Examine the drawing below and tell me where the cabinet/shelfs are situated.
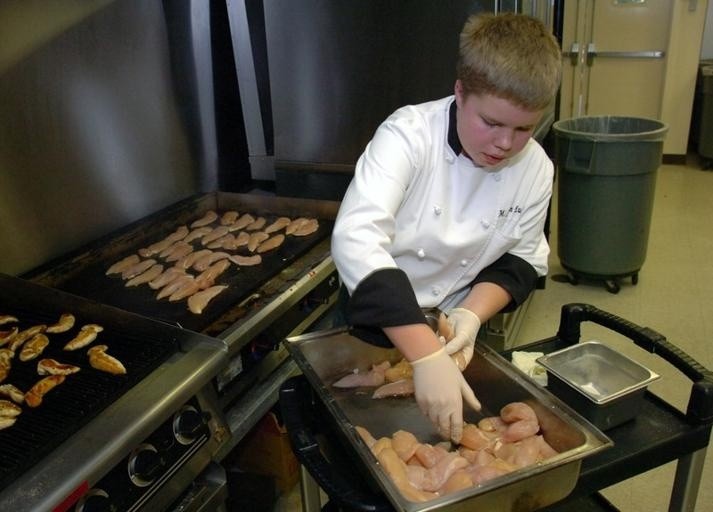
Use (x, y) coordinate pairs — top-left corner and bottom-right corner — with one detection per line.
(276, 299), (712, 510)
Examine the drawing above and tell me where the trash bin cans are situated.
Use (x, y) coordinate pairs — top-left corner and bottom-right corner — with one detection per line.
(551, 114), (668, 294)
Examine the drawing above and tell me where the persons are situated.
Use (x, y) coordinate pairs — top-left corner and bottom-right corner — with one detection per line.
(329, 12), (562, 443)
